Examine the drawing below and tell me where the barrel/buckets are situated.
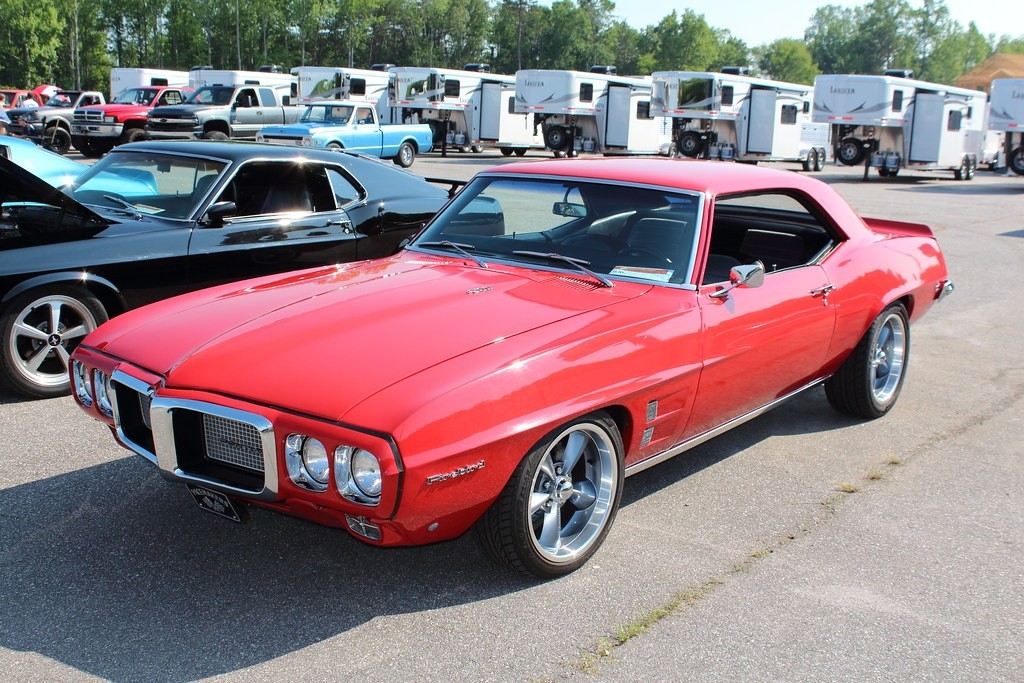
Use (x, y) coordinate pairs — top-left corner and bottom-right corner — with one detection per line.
(445, 132), (465, 145)
(574, 137), (593, 152)
(710, 145), (734, 159)
(871, 151), (898, 169)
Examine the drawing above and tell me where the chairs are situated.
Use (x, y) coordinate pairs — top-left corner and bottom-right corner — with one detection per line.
(734, 229), (804, 274)
(190, 173), (236, 204)
(626, 217), (688, 265)
(259, 179), (313, 213)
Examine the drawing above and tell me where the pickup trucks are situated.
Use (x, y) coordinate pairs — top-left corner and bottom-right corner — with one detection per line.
(256, 101), (432, 168)
(144, 84), (332, 141)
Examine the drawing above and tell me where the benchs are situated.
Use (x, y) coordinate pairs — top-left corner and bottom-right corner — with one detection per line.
(586, 211), (828, 272)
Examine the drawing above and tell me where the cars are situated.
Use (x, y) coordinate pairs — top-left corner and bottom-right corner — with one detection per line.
(1, 135), (159, 197)
(0, 140), (504, 399)
(69, 156), (955, 579)
(0, 90), (70, 111)
(70, 86), (201, 158)
(3, 91), (107, 153)
